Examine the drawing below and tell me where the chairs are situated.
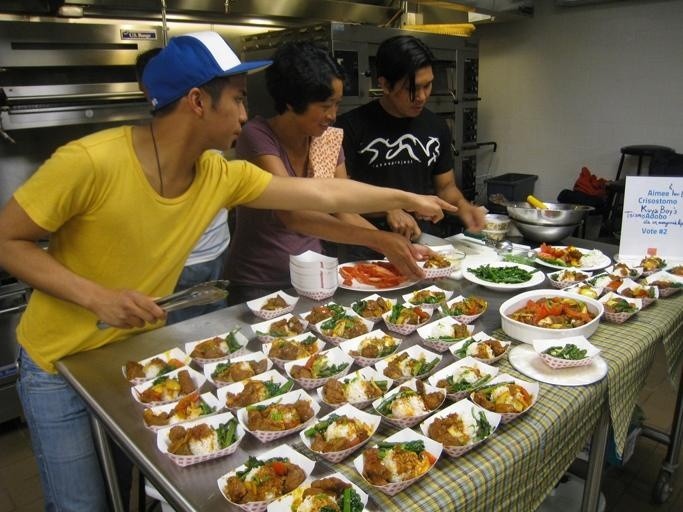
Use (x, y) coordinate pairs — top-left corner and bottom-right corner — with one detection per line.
(597, 144), (676, 245)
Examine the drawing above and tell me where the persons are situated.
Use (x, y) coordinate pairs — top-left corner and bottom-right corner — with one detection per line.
(0, 32), (458, 512)
(335, 35), (485, 242)
(148, 120), (163, 197)
(135, 48), (231, 326)
(224, 41), (442, 306)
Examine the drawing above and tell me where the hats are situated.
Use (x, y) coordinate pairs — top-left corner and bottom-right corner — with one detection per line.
(141, 31), (274, 110)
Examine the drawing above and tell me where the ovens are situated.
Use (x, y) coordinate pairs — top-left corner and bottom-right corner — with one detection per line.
(240, 22), (479, 236)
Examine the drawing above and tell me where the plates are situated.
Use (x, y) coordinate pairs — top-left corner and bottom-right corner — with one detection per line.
(463, 262), (546, 292)
(338, 260), (424, 292)
(528, 246), (611, 271)
(508, 343), (608, 386)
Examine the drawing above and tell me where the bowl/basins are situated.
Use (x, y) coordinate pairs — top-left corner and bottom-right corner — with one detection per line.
(501, 201), (595, 225)
(481, 230), (511, 242)
(508, 217), (584, 244)
(485, 214), (511, 230)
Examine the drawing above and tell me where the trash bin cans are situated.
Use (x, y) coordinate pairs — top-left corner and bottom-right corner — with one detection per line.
(484, 173), (538, 213)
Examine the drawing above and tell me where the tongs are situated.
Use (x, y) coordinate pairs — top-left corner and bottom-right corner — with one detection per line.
(97, 280), (230, 331)
